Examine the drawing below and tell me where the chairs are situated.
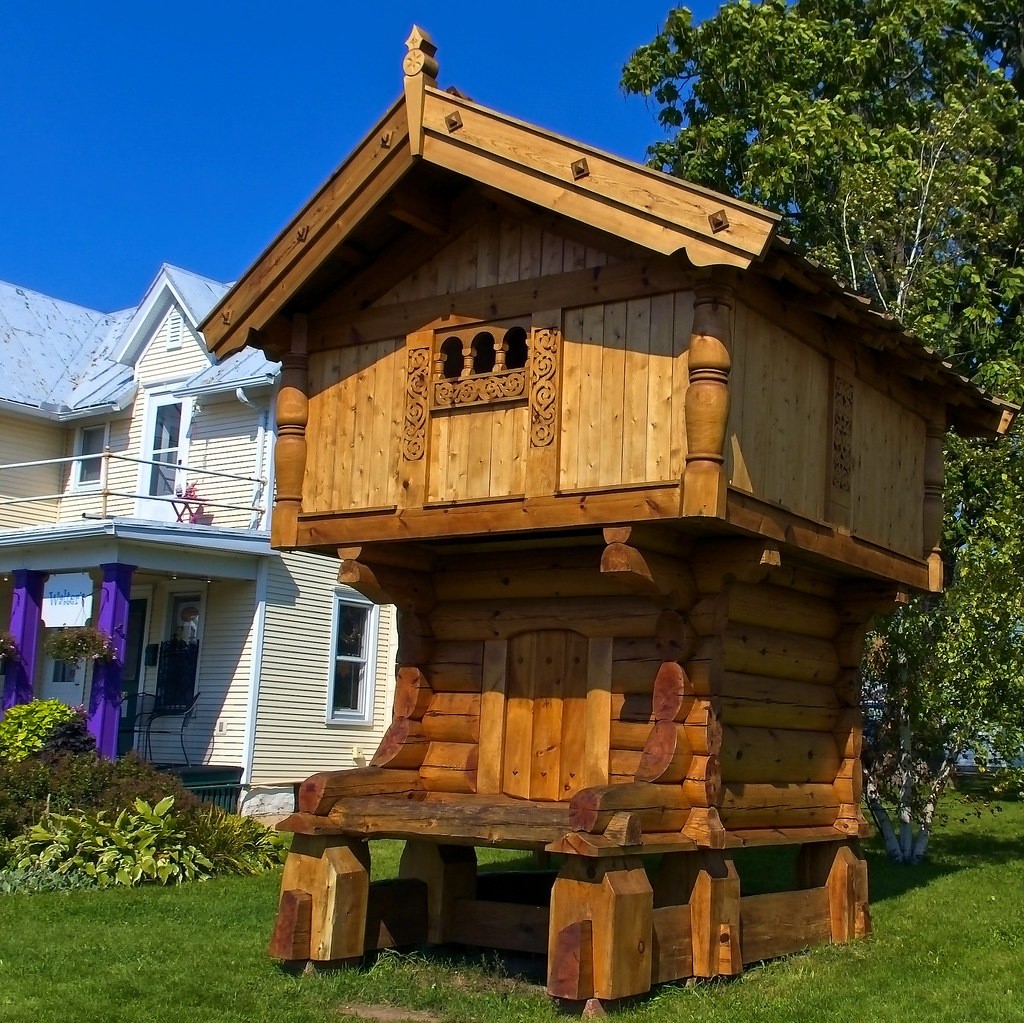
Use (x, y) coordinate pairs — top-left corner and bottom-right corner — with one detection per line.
(143, 691), (203, 767)
(117, 691), (157, 765)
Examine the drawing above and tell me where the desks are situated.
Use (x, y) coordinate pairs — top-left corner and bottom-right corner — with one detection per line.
(165, 498), (208, 528)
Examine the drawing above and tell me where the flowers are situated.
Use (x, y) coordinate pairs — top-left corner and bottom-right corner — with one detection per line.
(43, 627), (118, 663)
(0, 631), (18, 660)
